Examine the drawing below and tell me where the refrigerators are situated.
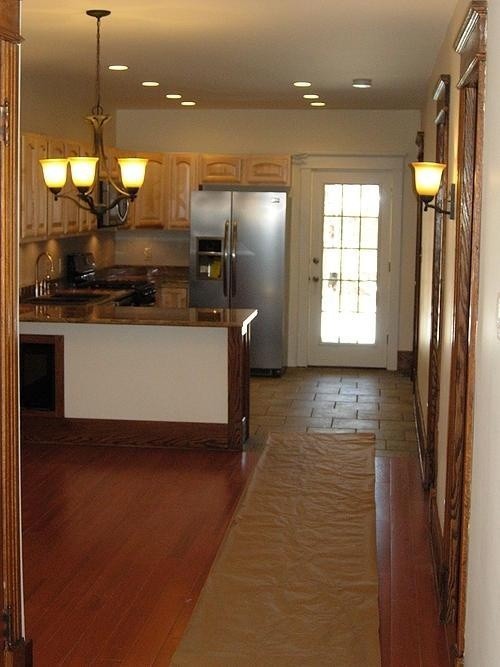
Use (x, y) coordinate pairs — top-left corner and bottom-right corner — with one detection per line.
(188, 189), (289, 378)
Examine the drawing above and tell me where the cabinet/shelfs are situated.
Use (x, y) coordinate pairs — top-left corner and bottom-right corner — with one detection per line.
(118, 151), (167, 230)
(65, 139), (120, 236)
(20, 135), (63, 244)
(167, 153), (200, 232)
(201, 153), (291, 185)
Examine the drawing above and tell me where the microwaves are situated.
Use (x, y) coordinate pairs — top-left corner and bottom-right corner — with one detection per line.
(100, 181), (129, 227)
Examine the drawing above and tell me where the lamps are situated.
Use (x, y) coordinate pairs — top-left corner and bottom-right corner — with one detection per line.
(38, 10), (148, 228)
(409, 160), (455, 219)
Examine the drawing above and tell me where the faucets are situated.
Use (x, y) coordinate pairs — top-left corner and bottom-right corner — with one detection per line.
(34, 252), (55, 296)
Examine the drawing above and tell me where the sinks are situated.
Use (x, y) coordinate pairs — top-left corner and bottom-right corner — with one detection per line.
(22, 293), (111, 305)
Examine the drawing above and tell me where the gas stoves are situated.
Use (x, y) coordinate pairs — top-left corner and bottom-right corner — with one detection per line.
(78, 279), (157, 304)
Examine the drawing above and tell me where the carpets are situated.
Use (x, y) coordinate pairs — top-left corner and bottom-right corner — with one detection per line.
(165, 432), (383, 665)
